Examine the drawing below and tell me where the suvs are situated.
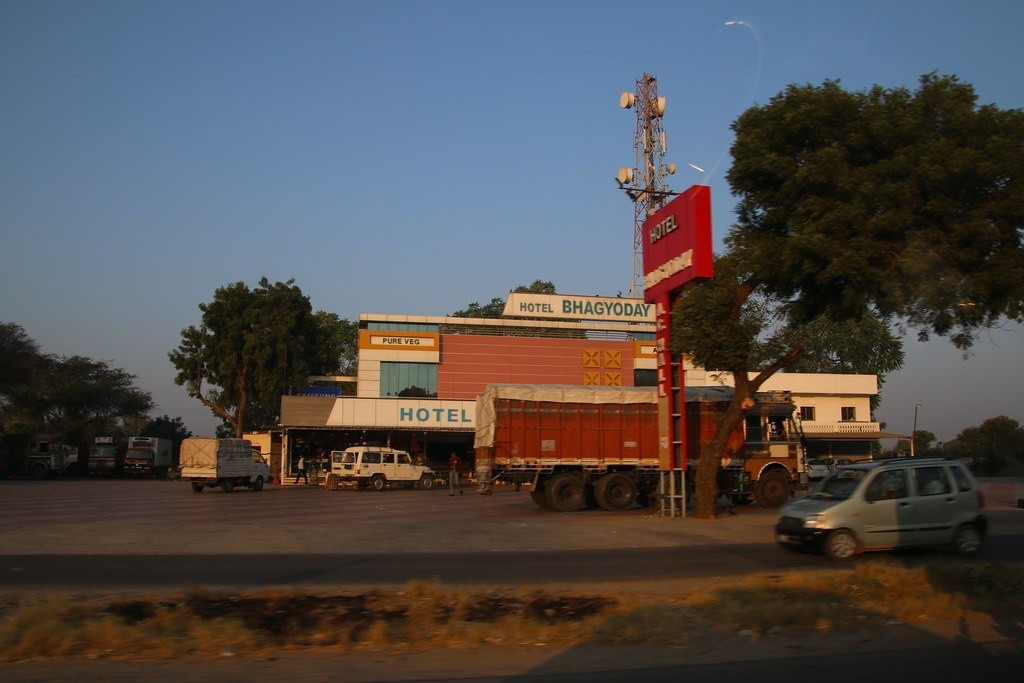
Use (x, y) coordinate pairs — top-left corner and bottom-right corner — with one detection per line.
(330, 446), (434, 492)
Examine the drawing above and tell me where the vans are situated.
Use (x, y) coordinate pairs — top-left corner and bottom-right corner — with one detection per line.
(775, 455), (990, 561)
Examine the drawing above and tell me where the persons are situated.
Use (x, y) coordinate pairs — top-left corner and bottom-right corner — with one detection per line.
(448, 452), (464, 497)
(295, 454), (309, 484)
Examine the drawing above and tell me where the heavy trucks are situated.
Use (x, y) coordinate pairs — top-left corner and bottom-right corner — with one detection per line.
(473, 380), (808, 514)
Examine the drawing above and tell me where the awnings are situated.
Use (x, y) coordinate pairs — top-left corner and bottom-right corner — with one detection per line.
(802, 432), (916, 459)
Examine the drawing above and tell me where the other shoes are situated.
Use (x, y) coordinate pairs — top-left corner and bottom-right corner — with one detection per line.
(460, 489), (463, 494)
(450, 494), (454, 496)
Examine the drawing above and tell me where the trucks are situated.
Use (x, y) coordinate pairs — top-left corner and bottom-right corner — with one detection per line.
(179, 437), (271, 493)
(88, 437), (121, 478)
(124, 436), (173, 479)
(21, 441), (78, 475)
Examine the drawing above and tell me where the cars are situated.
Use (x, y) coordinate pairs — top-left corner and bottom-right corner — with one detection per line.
(807, 457), (859, 480)
